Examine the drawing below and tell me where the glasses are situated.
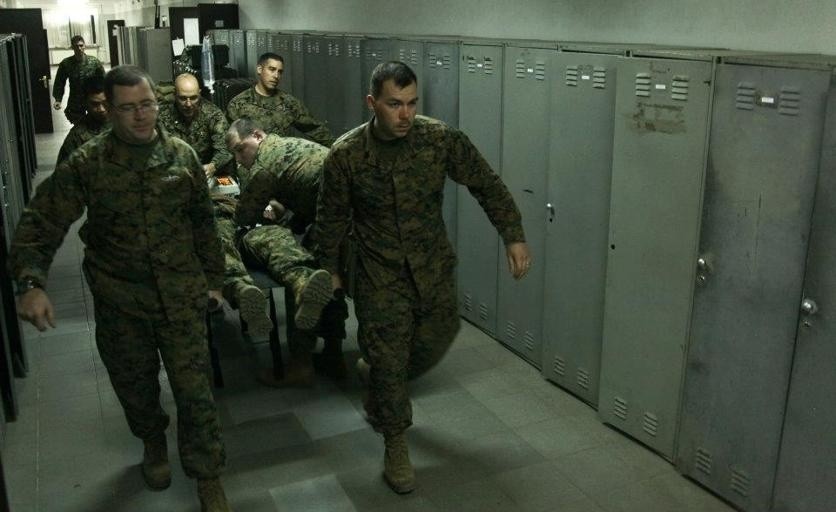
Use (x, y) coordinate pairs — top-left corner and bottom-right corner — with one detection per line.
(110, 101), (160, 114)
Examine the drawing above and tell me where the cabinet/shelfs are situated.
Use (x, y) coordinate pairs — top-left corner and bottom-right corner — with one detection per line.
(0, 5), (60, 504)
(205, 26), (836, 509)
(115, 26), (172, 85)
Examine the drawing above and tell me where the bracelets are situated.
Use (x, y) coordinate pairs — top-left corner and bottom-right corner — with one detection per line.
(265, 204), (275, 211)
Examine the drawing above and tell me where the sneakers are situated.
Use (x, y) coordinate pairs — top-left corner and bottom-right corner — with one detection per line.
(292, 269), (335, 331)
(233, 281), (273, 337)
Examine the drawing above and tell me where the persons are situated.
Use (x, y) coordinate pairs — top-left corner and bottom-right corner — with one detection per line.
(225, 52), (337, 148)
(54, 82), (110, 165)
(6, 66), (228, 511)
(51, 33), (104, 124)
(157, 70), (232, 177)
(225, 115), (375, 384)
(210, 192), (336, 348)
(310, 60), (532, 495)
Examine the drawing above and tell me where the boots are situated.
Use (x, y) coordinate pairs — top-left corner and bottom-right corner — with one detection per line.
(141, 432), (172, 491)
(196, 476), (229, 511)
(384, 432), (417, 495)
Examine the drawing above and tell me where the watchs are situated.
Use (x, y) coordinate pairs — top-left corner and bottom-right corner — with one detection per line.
(17, 280), (45, 293)
